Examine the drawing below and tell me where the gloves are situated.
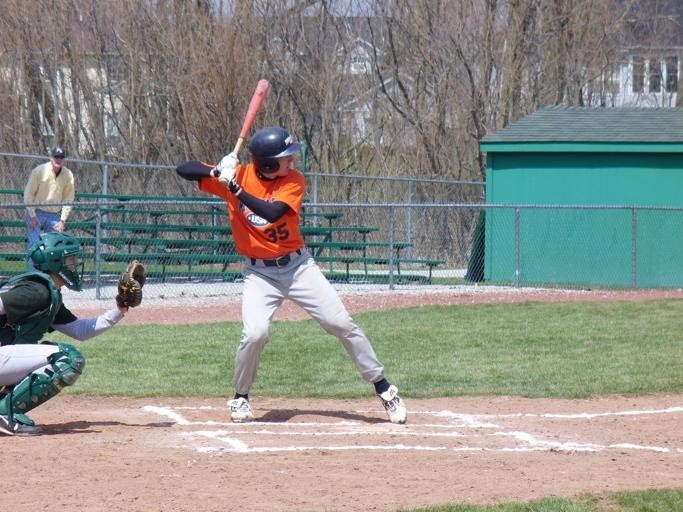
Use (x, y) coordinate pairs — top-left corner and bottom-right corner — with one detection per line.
(210, 152), (240, 192)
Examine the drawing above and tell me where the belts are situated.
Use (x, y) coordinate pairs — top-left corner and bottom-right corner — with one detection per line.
(251, 249), (303, 267)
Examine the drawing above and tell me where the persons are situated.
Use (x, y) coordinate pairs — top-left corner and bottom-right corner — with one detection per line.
(174, 124), (407, 424)
(23, 146), (76, 272)
(0, 231), (146, 436)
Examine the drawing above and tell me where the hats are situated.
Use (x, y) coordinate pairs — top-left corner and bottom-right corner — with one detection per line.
(53, 148), (65, 157)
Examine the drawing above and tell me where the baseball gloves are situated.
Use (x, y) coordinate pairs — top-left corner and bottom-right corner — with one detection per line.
(116, 261), (145, 308)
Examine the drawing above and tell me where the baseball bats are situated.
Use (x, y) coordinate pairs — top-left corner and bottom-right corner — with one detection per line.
(219, 80), (272, 186)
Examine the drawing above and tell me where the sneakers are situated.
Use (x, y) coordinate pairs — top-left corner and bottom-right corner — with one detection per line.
(0, 414), (43, 436)
(228, 397), (254, 424)
(375, 383), (407, 423)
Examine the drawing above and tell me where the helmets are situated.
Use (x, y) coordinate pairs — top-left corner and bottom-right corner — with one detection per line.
(25, 232), (84, 292)
(248, 128), (302, 173)
